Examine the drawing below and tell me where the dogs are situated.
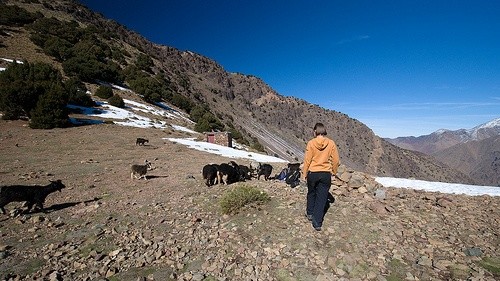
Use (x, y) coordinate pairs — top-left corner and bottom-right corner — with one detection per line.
(136, 138), (149, 146)
(202, 160), (301, 187)
(0, 180), (67, 216)
(131, 159), (153, 181)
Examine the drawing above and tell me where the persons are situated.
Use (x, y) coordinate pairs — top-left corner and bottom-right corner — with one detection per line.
(303, 123), (339, 230)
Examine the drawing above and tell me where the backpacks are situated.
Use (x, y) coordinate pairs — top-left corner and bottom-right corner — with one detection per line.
(286, 170), (301, 188)
(278, 168), (290, 180)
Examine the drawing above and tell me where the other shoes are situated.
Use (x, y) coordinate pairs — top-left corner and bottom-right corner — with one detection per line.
(312, 221), (321, 230)
(305, 210), (313, 221)
